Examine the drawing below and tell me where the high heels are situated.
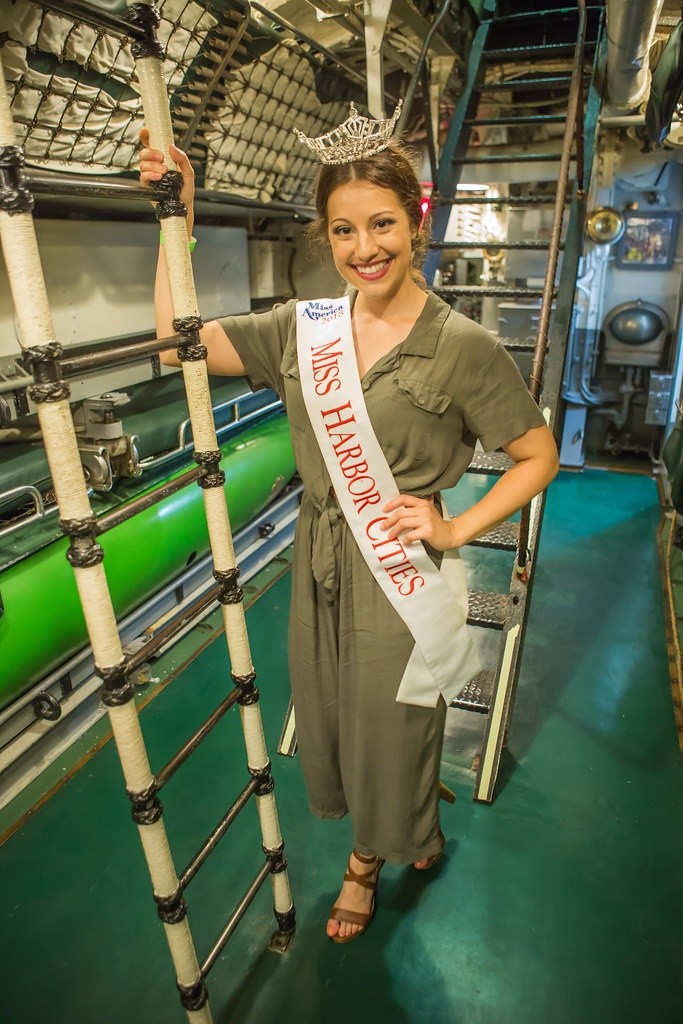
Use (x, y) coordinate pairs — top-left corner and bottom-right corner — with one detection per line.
(415, 782), (456, 868)
(330, 848), (385, 944)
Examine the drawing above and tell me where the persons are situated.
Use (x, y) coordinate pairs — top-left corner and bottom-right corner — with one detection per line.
(136, 99), (560, 943)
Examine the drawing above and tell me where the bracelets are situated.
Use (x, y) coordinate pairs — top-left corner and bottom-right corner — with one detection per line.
(159, 227), (197, 254)
